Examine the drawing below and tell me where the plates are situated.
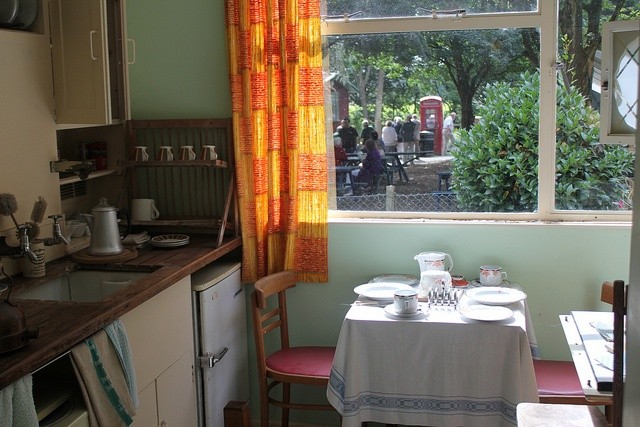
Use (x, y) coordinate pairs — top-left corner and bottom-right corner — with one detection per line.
(354, 282), (414, 301)
(373, 274), (419, 285)
(466, 286), (528, 305)
(458, 305), (512, 321)
(151, 235), (190, 248)
(470, 277), (511, 287)
(385, 305), (422, 317)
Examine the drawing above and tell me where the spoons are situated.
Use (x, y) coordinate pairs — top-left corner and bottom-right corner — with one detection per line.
(350, 302), (386, 309)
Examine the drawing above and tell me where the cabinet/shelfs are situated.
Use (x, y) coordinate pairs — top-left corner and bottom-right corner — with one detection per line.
(31, 0), (131, 123)
(55, 129), (125, 222)
(129, 348), (199, 427)
(115, 118), (239, 248)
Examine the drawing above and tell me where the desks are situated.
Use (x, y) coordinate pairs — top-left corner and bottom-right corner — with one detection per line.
(336, 151), (434, 182)
(336, 165), (361, 195)
(436, 172), (451, 209)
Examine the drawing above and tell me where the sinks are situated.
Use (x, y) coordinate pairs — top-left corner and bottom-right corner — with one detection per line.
(9, 261), (165, 302)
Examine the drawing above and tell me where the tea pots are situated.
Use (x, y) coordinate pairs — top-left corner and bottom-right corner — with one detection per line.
(414, 251), (453, 294)
(77, 197), (125, 255)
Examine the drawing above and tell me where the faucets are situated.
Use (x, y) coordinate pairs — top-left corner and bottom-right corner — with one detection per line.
(0, 223), (39, 263)
(36, 214), (70, 247)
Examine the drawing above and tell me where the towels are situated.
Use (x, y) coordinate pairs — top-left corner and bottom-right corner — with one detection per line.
(69, 329), (136, 427)
(102, 318), (139, 408)
(0, 373), (39, 427)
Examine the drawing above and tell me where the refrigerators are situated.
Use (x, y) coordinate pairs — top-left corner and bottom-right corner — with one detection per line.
(192, 264), (249, 426)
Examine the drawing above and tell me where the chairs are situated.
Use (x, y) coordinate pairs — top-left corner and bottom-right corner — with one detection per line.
(532, 281), (626, 424)
(250, 270), (342, 427)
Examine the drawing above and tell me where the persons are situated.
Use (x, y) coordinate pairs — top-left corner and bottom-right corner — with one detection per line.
(443, 112), (458, 153)
(353, 139), (383, 195)
(398, 115), (416, 164)
(333, 136), (348, 196)
(381, 121), (398, 155)
(412, 114), (424, 159)
(392, 117), (398, 125)
(336, 117), (358, 152)
(363, 131), (384, 152)
(360, 119), (377, 146)
(394, 119), (404, 150)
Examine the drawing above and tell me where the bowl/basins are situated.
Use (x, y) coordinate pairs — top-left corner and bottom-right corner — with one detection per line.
(65, 219), (87, 238)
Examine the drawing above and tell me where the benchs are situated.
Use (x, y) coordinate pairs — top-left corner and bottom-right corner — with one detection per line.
(386, 157), (410, 182)
(337, 157), (394, 184)
(336, 174), (383, 196)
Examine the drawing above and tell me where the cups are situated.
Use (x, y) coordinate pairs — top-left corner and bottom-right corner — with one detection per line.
(21, 241), (46, 278)
(421, 272), (451, 296)
(134, 146), (150, 161)
(480, 265), (507, 286)
(394, 289), (418, 314)
(180, 146), (196, 161)
(131, 198), (159, 222)
(202, 145), (217, 160)
(159, 146), (174, 161)
